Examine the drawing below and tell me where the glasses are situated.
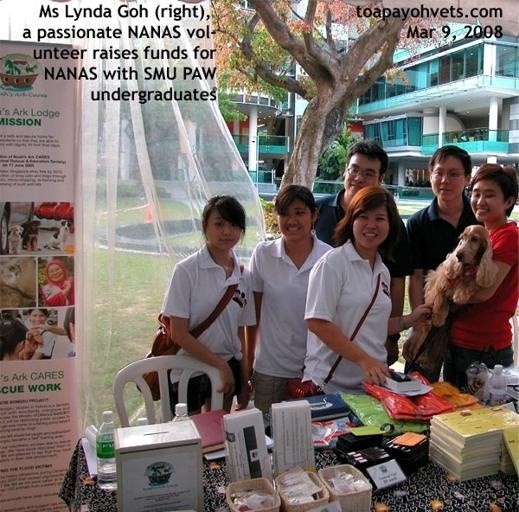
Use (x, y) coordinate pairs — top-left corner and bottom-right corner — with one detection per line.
(346, 166), (381, 179)
(431, 170), (466, 178)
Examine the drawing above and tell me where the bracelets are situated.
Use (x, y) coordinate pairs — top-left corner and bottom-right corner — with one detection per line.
(398, 317), (404, 330)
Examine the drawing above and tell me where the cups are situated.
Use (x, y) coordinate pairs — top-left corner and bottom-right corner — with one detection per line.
(465, 358), (490, 403)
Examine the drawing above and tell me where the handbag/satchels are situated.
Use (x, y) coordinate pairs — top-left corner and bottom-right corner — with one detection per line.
(286, 377), (319, 401)
(135, 312), (182, 402)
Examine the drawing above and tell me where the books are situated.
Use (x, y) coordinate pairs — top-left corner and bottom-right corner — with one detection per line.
(429, 399), (517, 488)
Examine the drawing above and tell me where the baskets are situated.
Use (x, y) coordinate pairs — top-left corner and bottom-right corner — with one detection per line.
(272, 469), (330, 511)
(224, 477), (281, 511)
(317, 463), (373, 511)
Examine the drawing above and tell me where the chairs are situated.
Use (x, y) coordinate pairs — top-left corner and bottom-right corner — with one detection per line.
(109, 355), (223, 426)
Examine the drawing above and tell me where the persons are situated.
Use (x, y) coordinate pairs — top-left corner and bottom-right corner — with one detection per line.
(436, 161), (518, 389)
(246, 182), (337, 411)
(398, 143), (489, 361)
(40, 260), (74, 306)
(299, 186), (429, 400)
(158, 195), (258, 415)
(0, 307), (76, 362)
(310, 138), (408, 368)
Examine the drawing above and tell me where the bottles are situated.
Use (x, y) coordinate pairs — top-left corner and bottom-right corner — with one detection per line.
(171, 402), (191, 423)
(488, 364), (508, 407)
(96, 410), (119, 491)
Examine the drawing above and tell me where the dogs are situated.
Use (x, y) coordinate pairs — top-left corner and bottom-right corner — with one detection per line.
(3, 329), (43, 360)
(21, 220), (42, 251)
(8, 225), (25, 255)
(43, 220), (72, 251)
(409, 224), (496, 356)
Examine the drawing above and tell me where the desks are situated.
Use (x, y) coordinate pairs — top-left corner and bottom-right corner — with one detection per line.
(77, 385), (519, 512)
